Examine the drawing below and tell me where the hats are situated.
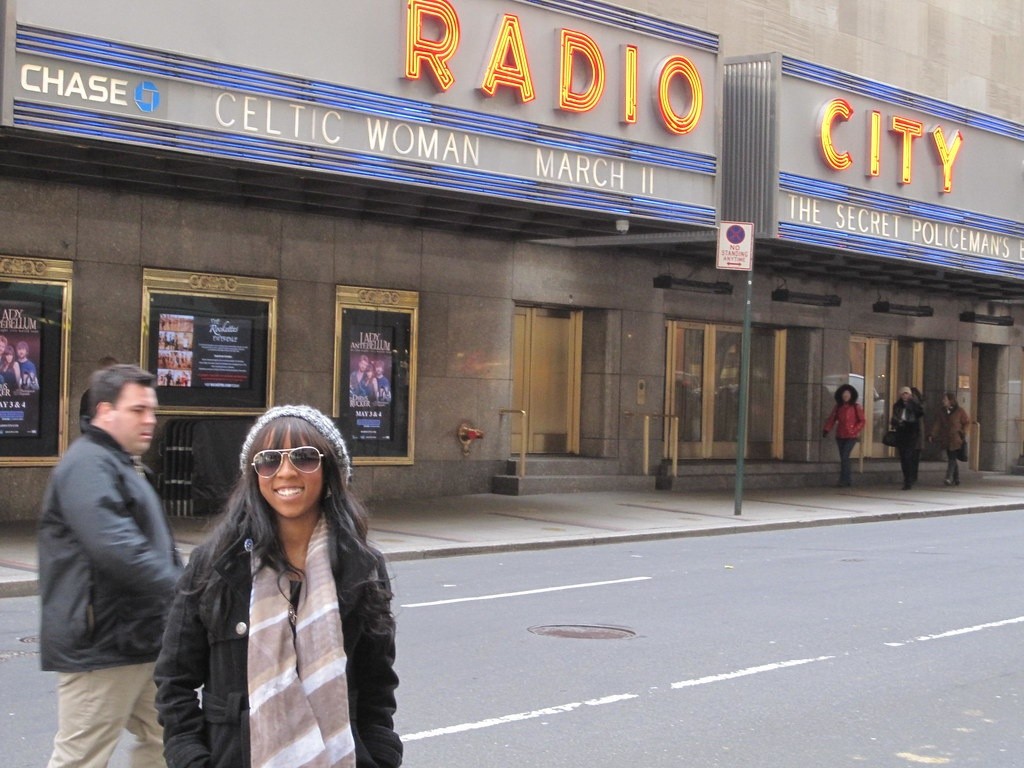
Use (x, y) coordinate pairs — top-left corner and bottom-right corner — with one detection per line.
(899, 384), (911, 396)
(240, 404), (351, 487)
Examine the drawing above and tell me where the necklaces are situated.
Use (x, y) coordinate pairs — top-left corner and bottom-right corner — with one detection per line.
(286, 602), (298, 626)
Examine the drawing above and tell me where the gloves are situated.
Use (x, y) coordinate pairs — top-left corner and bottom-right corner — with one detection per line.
(823, 429), (828, 439)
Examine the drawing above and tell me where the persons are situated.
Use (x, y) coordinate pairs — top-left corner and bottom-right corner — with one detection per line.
(823, 384), (865, 486)
(158, 319), (188, 386)
(928, 393), (970, 486)
(890, 386), (925, 490)
(154, 404), (405, 768)
(349, 355), (391, 407)
(39, 364), (185, 768)
(80, 356), (119, 433)
(0, 335), (37, 390)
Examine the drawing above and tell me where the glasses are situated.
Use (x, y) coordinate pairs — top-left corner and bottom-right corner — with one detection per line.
(251, 446), (324, 480)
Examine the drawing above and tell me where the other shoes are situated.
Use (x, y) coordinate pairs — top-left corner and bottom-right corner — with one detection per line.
(945, 478), (960, 486)
(902, 481), (912, 491)
(836, 480), (852, 487)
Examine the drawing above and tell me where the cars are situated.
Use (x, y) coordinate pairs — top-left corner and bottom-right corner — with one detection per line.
(677, 371), (769, 400)
(822, 375), (886, 419)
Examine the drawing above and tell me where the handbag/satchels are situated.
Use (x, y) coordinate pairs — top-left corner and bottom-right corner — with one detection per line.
(956, 440), (968, 462)
(883, 422), (900, 448)
